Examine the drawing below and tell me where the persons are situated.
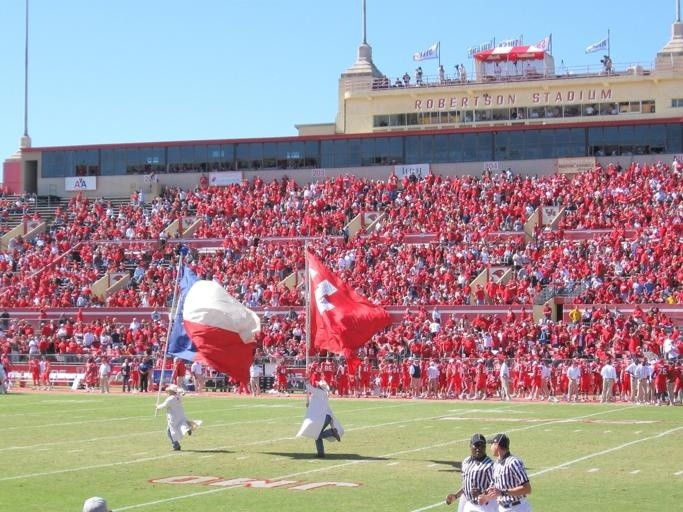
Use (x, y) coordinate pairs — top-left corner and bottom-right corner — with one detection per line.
(494, 64), (502, 80)
(600, 55), (613, 75)
(446, 434), (496, 512)
(373, 68), (423, 86)
(83, 496), (109, 510)
(296, 372), (343, 459)
(438, 64), (467, 84)
(488, 434), (532, 512)
(463, 102), (619, 121)
(155, 384), (200, 452)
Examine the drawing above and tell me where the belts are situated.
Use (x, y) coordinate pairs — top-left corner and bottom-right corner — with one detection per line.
(471, 500), (488, 506)
(503, 501), (521, 508)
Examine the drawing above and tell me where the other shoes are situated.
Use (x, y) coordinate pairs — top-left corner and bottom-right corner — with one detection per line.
(332, 392), (682, 408)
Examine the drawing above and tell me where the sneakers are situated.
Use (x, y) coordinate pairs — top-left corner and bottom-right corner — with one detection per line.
(315, 454), (325, 458)
(333, 428), (340, 442)
(33, 387), (150, 393)
(195, 389), (307, 397)
(187, 430), (191, 435)
(170, 447), (181, 451)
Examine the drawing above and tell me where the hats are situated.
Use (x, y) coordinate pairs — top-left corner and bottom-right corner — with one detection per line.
(489, 434), (510, 447)
(310, 304), (683, 370)
(470, 434), (486, 445)
(165, 384), (178, 392)
(315, 380), (329, 390)
(82, 496), (113, 512)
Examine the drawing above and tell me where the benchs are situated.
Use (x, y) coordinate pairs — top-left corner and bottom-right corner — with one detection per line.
(0, 191), (195, 307)
(4, 368), (87, 393)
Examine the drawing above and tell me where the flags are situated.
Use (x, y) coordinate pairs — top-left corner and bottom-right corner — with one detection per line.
(586, 36), (609, 54)
(413, 43), (438, 61)
(467, 40), (494, 57)
(499, 38), (520, 47)
(536, 36), (549, 50)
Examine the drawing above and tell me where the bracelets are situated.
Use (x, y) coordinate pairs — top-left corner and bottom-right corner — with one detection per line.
(455, 494), (458, 500)
(501, 489), (508, 497)
